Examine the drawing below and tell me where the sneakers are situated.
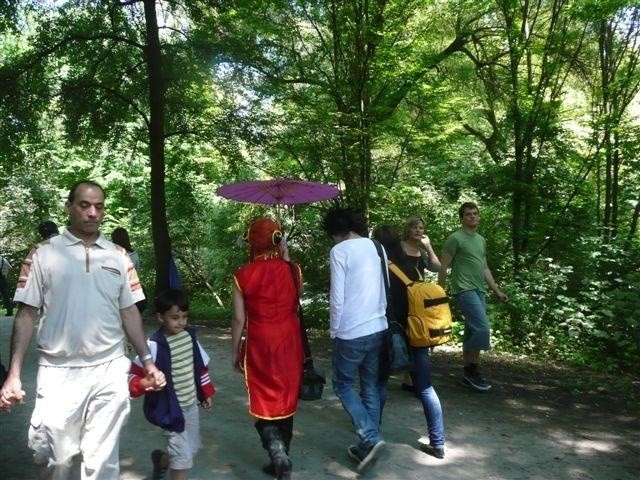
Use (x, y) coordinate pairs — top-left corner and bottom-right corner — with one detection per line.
(355, 439), (387, 476)
(401, 381), (416, 392)
(152, 447), (170, 480)
(347, 443), (361, 462)
(460, 361), (493, 391)
(420, 443), (446, 458)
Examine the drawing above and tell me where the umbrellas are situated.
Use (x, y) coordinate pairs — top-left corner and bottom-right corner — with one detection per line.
(216, 179), (339, 230)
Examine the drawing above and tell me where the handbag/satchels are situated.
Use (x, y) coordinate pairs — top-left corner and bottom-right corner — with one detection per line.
(385, 317), (414, 371)
(297, 361), (326, 401)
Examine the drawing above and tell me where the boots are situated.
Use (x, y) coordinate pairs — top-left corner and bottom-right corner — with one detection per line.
(253, 416), (293, 480)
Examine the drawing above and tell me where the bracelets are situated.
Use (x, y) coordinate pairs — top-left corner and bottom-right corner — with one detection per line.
(139, 354), (153, 363)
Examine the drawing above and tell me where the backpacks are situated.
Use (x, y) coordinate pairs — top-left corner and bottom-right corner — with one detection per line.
(388, 258), (454, 347)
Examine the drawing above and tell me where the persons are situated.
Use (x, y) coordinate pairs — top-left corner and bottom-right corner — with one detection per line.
(323, 207), (390, 474)
(38, 221), (60, 241)
(112, 226), (139, 274)
(128, 288), (216, 479)
(0, 179), (162, 479)
(373, 226), (445, 457)
(0, 251), (14, 316)
(399, 217), (441, 393)
(439, 202), (507, 391)
(230, 219), (304, 479)
(0, 360), (25, 415)
